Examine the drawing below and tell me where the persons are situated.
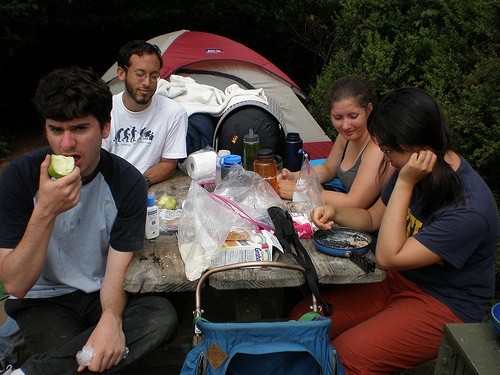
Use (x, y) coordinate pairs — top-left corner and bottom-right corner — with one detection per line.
(277, 76), (397, 210)
(100, 40), (188, 190)
(287, 88), (500, 375)
(0, 66), (180, 375)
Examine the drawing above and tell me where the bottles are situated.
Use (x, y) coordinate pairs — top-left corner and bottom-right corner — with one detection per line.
(253, 149), (278, 194)
(215, 150), (230, 186)
(144, 196), (159, 239)
(219, 155), (243, 182)
(283, 133), (303, 172)
(244, 129), (260, 171)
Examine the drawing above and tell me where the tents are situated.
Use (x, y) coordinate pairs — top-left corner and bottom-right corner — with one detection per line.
(101, 29), (334, 161)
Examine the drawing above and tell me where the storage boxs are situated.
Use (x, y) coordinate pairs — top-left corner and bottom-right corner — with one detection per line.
(209, 229), (281, 269)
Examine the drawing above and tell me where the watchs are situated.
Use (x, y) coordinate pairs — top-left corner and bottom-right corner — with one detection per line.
(143, 175), (150, 189)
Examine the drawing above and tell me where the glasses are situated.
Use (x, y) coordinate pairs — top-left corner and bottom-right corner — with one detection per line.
(379, 141), (404, 155)
(124, 64), (162, 83)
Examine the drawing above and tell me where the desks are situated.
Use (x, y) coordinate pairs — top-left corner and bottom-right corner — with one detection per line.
(122, 167), (387, 324)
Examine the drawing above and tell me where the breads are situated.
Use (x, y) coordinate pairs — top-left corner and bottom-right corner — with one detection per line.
(225, 231), (247, 241)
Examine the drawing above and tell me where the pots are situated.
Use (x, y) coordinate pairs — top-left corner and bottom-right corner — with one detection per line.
(312, 227), (376, 274)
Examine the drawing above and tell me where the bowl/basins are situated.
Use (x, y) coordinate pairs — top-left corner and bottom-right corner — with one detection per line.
(490, 302), (500, 337)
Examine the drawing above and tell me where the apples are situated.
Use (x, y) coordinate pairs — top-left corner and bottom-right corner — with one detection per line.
(156, 195), (176, 210)
(48, 154), (75, 179)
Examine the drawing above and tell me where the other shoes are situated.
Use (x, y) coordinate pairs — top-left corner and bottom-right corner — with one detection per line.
(0, 330), (25, 370)
